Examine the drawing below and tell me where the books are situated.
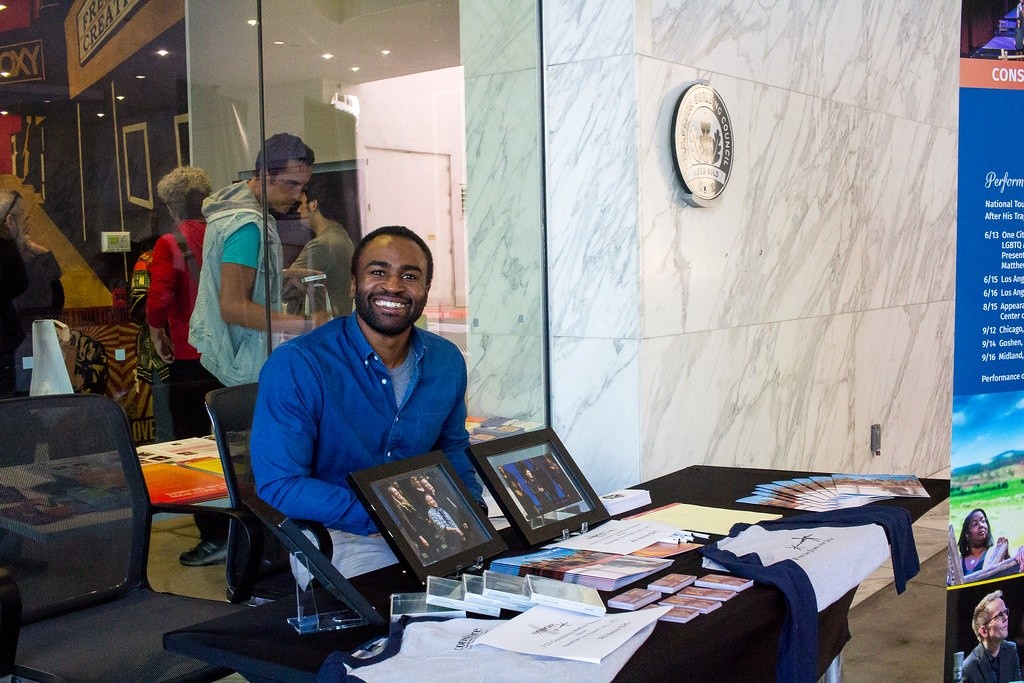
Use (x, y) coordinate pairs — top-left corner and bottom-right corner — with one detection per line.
(465, 415), (545, 445)
(0, 429), (251, 539)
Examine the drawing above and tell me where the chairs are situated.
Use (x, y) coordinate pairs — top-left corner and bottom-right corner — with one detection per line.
(0, 393), (258, 683)
(204, 382), (336, 605)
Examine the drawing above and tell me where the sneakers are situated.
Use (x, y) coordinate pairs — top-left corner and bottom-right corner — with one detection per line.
(178, 539), (230, 566)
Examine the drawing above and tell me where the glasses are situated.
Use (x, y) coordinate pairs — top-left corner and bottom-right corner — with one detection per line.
(983, 608), (1010, 626)
(967, 518), (988, 529)
(2, 189), (24, 222)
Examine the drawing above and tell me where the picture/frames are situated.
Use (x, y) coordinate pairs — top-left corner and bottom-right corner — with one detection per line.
(344, 449), (510, 587)
(464, 426), (612, 548)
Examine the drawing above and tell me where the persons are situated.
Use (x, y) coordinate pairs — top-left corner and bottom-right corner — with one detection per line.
(947, 509), (1020, 587)
(128, 132), (356, 565)
(0, 192), (65, 683)
(388, 474), (479, 561)
(496, 456), (581, 518)
(247, 226), (487, 592)
(962, 589), (1021, 683)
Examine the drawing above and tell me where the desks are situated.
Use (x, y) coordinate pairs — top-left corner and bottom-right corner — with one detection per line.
(161, 465), (950, 683)
